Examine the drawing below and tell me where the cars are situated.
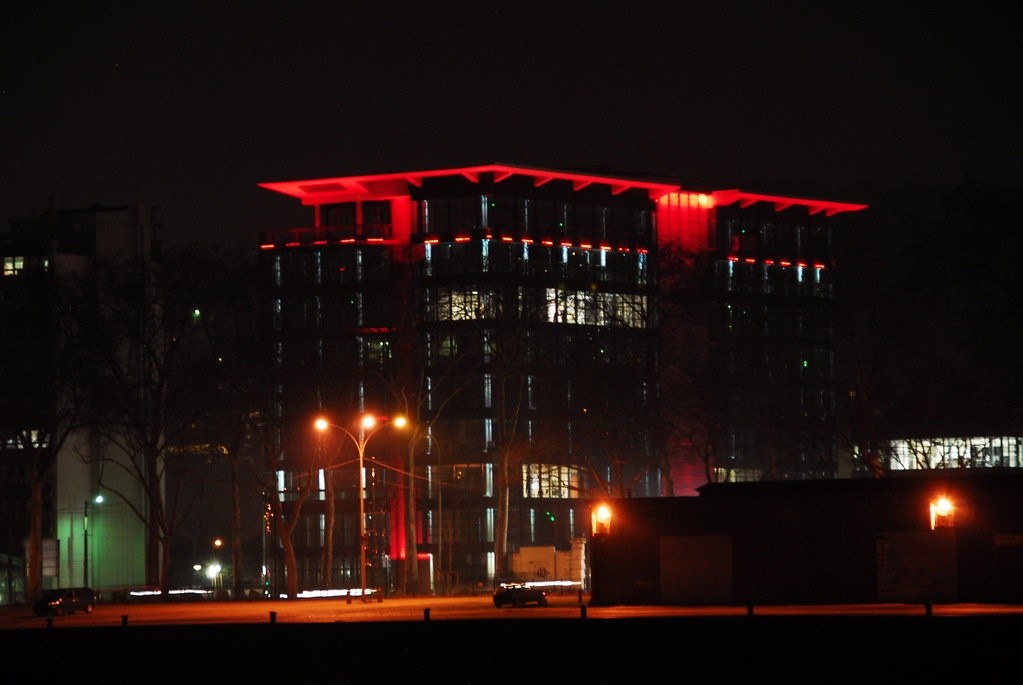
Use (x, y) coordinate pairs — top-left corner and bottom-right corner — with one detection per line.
(492, 585), (546, 609)
(33, 587), (98, 618)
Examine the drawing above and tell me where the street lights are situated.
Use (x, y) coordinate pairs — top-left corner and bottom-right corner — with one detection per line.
(591, 504), (611, 534)
(84, 495), (102, 592)
(315, 412), (410, 598)
(927, 499), (954, 531)
